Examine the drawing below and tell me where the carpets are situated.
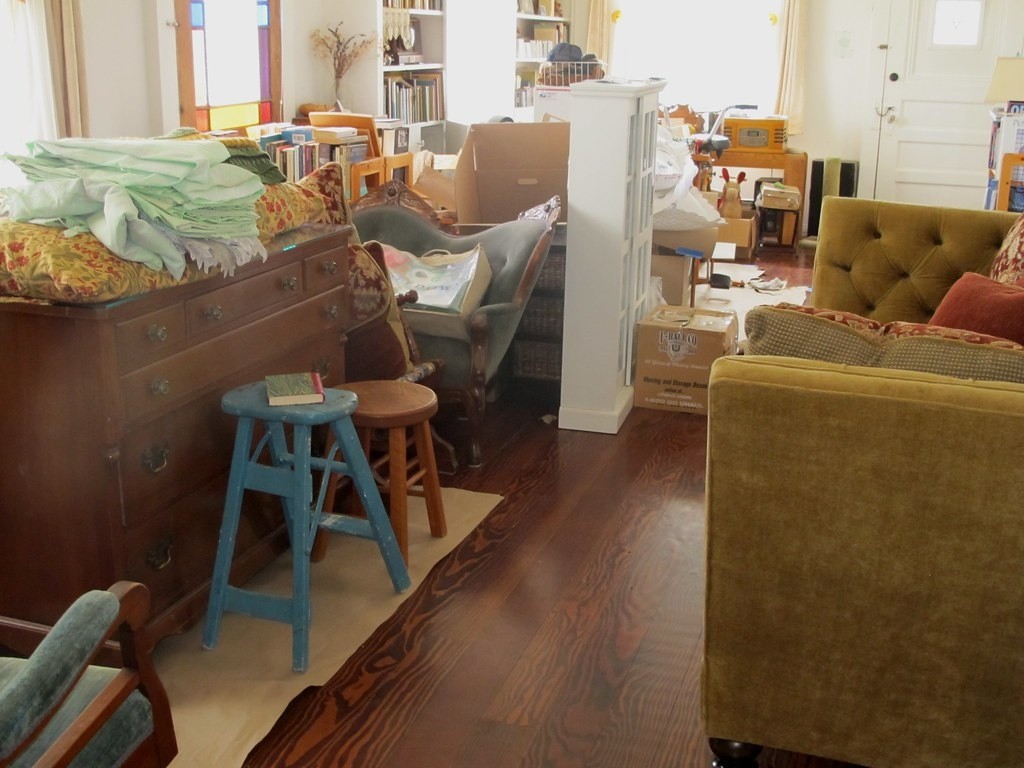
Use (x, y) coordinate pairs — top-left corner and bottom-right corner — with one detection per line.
(150, 485), (504, 768)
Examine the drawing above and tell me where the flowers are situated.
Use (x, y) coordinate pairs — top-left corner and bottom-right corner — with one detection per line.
(310, 17), (376, 102)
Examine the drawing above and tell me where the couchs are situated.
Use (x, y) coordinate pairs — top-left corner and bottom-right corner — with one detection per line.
(700, 194), (1024, 768)
(349, 179), (561, 471)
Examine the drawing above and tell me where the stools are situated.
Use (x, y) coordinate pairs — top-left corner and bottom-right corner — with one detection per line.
(310, 380), (447, 570)
(200, 380), (413, 675)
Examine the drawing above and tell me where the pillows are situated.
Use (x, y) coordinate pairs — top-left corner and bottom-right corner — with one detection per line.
(745, 208), (1024, 384)
(0, 156), (416, 380)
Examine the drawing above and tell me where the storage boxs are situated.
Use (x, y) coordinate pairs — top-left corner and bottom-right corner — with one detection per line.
(651, 255), (692, 307)
(633, 306), (740, 416)
(714, 209), (759, 262)
(449, 122), (570, 235)
(757, 181), (801, 210)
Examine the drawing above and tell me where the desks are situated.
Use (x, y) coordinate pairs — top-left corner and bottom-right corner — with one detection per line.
(709, 148), (808, 251)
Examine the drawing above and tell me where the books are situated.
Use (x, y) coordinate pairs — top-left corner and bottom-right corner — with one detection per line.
(514, 342), (561, 379)
(265, 372), (325, 406)
(516, 69), (536, 107)
(258, 124), (368, 200)
(383, 0), (442, 11)
(384, 19), (422, 64)
(384, 72), (445, 125)
(517, 22), (567, 58)
(519, 297), (564, 338)
(536, 254), (565, 296)
(517, 0), (555, 16)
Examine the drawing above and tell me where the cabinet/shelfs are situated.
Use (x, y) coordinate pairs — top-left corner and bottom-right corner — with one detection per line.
(987, 153), (1024, 212)
(0, 224), (354, 670)
(376, 0), (448, 157)
(514, 0), (574, 109)
(346, 153), (413, 210)
(558, 77), (669, 434)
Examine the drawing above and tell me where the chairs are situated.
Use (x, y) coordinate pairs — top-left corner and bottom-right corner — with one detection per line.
(0, 583), (178, 768)
